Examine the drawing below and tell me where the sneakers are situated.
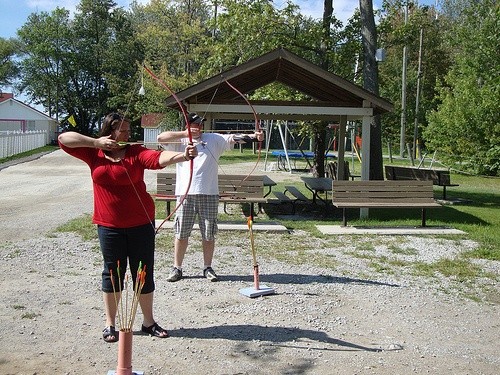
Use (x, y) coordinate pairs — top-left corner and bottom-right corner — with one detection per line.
(203, 267), (217, 280)
(166, 267), (182, 282)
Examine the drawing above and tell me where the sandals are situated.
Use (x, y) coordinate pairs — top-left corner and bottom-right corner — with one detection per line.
(102, 326), (117, 342)
(142, 323), (168, 338)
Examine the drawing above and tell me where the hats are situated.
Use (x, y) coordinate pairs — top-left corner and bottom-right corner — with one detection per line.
(185, 113), (208, 124)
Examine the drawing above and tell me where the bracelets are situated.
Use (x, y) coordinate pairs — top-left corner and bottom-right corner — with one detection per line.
(183, 154), (189, 161)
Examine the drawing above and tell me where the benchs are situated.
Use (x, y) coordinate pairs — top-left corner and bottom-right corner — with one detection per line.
(155, 173), (267, 223)
(332, 180), (442, 228)
(272, 191), (291, 213)
(285, 185), (310, 212)
(385, 165), (459, 199)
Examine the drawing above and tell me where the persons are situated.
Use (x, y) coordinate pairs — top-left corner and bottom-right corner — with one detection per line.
(58, 112), (198, 343)
(157, 112), (264, 282)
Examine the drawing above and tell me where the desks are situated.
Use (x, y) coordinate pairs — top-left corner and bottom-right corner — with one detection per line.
(260, 175), (277, 214)
(301, 177), (334, 209)
